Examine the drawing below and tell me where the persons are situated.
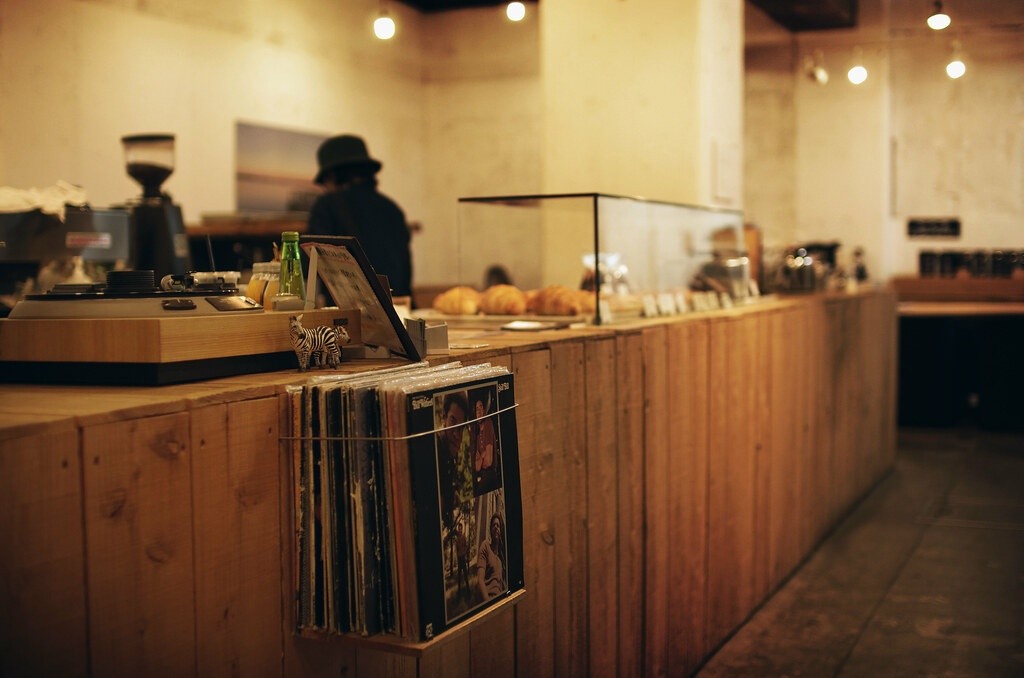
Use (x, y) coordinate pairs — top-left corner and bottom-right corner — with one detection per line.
(307, 135), (416, 308)
(456, 523), (472, 601)
(474, 398), (499, 496)
(475, 514), (504, 606)
(435, 390), (467, 515)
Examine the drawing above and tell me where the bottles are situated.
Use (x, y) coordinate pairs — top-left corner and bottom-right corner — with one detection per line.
(278, 232), (305, 300)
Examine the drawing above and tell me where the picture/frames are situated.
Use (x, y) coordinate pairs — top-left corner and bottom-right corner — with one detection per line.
(299, 234), (423, 364)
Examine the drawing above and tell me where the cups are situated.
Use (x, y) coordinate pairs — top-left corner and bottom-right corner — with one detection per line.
(246, 263), (280, 308)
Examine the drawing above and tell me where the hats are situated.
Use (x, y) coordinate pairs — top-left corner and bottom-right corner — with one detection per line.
(314, 135), (381, 185)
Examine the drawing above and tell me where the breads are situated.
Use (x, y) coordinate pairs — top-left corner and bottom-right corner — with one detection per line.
(434, 283), (598, 316)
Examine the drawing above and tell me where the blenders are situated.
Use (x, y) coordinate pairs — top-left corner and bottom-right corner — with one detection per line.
(123, 133), (192, 274)
